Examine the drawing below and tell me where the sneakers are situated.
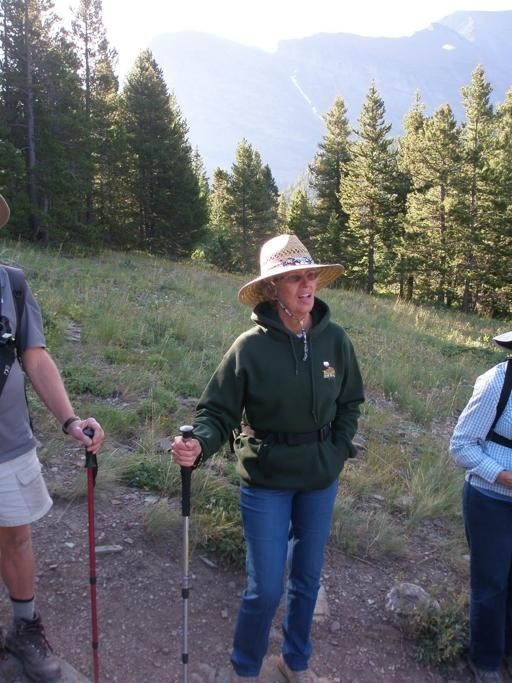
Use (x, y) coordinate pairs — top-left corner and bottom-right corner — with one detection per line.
(5, 611), (64, 682)
(277, 652), (320, 683)
(229, 668), (261, 683)
(467, 657), (504, 683)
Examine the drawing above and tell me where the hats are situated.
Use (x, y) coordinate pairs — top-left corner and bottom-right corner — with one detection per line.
(0, 194), (11, 229)
(492, 331), (512, 354)
(237, 234), (346, 308)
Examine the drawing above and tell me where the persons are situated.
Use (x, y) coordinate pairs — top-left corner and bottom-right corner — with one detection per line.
(166, 232), (368, 682)
(0, 193), (106, 682)
(445, 325), (512, 682)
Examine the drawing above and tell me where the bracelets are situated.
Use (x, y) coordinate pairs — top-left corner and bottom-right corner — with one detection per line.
(62, 415), (81, 434)
